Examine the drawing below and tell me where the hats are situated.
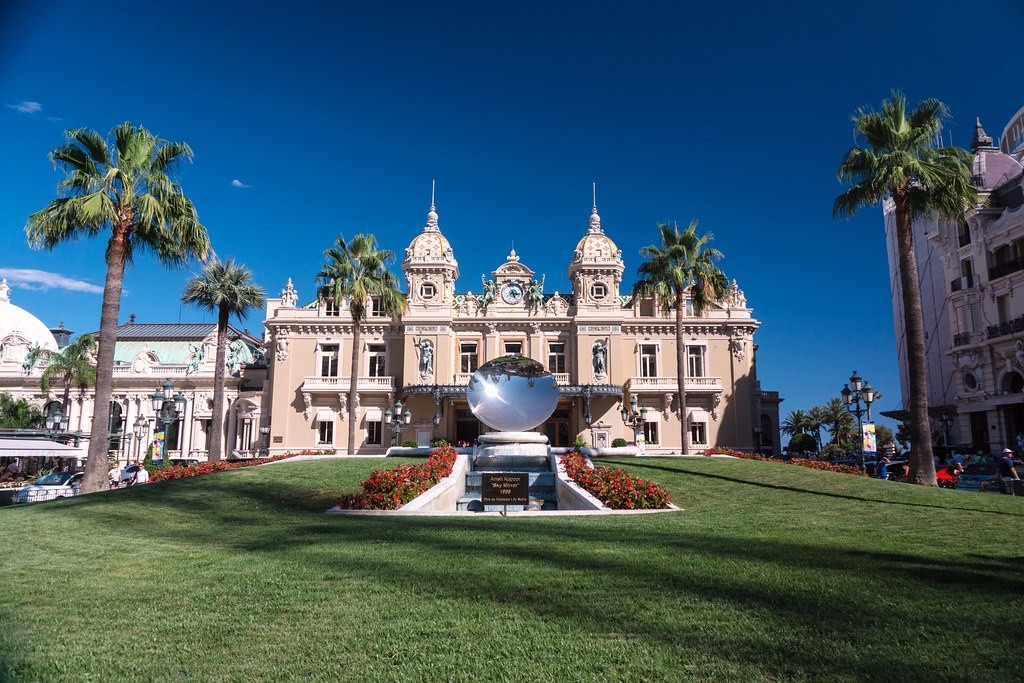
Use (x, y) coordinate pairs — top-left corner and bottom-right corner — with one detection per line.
(1002, 448), (1015, 453)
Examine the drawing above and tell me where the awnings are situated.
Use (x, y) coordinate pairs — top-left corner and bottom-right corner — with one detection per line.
(0, 440), (83, 456)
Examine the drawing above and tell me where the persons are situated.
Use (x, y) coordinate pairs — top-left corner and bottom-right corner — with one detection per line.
(480, 273), (496, 308)
(935, 462), (964, 486)
(107, 463), (122, 490)
(899, 459), (910, 479)
(932, 448), (988, 465)
(528, 274), (545, 308)
(418, 336), (435, 375)
(7, 459), (19, 481)
(130, 460), (150, 488)
(1017, 430), (1024, 454)
(998, 448), (1020, 495)
(875, 454), (891, 480)
(595, 341), (607, 374)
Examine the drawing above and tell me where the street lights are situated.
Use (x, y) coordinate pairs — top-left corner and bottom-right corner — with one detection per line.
(153, 377), (187, 466)
(841, 370), (875, 475)
(259, 424), (272, 457)
(133, 412), (149, 463)
(384, 399), (413, 446)
(43, 408), (67, 441)
(621, 397), (647, 446)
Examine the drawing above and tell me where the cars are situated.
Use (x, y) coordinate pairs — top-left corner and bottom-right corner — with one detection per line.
(11, 471), (84, 504)
(151, 458), (200, 473)
(119, 462), (139, 485)
(832, 450), (906, 475)
